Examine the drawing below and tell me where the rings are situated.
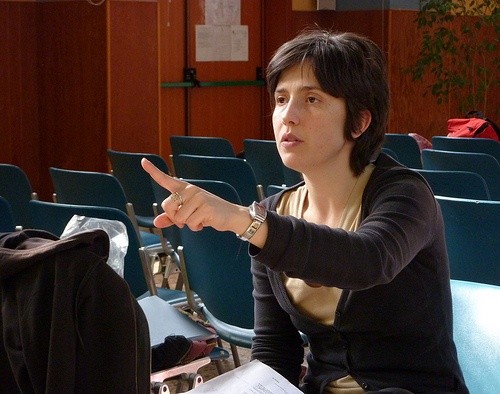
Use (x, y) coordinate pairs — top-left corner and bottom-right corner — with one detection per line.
(170, 191), (184, 205)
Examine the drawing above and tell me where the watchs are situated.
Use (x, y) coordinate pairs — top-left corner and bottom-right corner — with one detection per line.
(235, 201), (267, 242)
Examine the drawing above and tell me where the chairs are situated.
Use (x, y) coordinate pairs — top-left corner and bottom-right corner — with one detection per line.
(0, 134), (499, 394)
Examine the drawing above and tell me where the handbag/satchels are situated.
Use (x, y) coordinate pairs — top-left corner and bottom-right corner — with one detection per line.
(447, 111), (500, 143)
(0, 228), (151, 394)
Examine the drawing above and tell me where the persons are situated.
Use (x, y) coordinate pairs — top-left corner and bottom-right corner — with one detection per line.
(142, 31), (470, 394)
